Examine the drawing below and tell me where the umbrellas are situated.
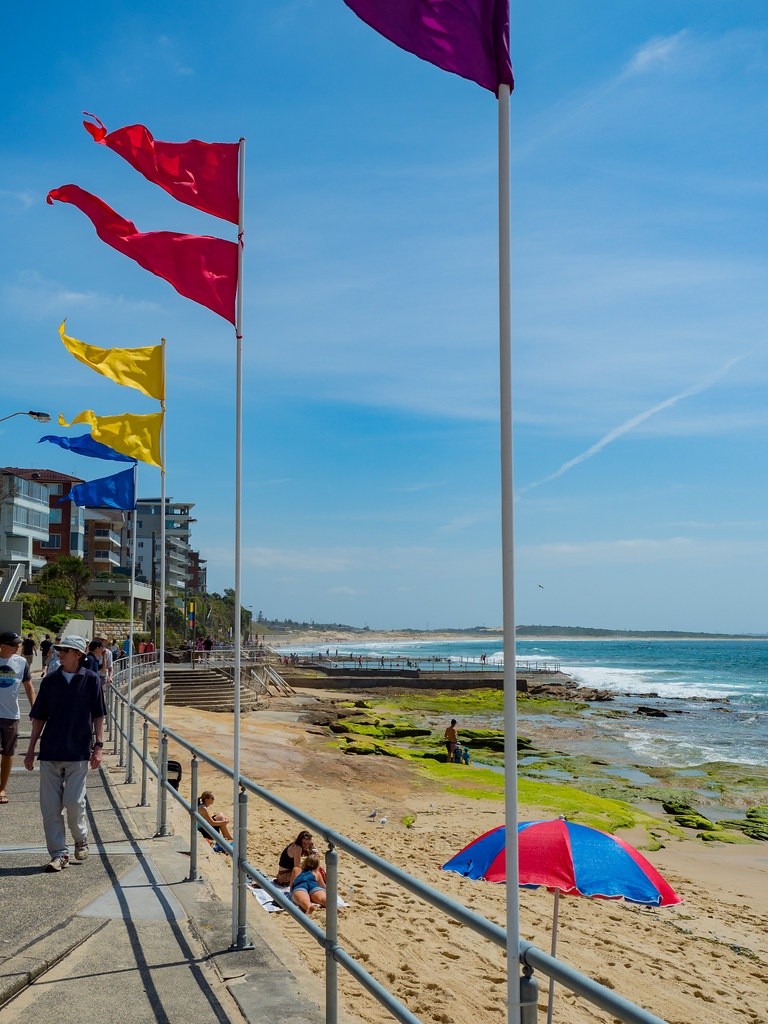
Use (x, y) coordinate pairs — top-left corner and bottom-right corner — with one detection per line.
(442, 815), (685, 1024)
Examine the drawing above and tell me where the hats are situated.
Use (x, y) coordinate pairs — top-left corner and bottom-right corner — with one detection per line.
(94, 633), (109, 643)
(0, 631), (24, 645)
(53, 635), (87, 655)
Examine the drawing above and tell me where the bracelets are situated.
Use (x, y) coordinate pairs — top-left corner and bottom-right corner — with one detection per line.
(93, 742), (103, 747)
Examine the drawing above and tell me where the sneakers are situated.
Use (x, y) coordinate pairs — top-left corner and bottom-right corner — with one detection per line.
(45, 856), (69, 871)
(74, 838), (89, 860)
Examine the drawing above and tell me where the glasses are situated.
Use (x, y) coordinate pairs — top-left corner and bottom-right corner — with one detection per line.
(57, 647), (79, 653)
(206, 797), (215, 800)
(3, 643), (19, 647)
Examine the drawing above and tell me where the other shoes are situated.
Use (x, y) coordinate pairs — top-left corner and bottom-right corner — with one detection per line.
(41, 672), (45, 677)
(0, 790), (8, 804)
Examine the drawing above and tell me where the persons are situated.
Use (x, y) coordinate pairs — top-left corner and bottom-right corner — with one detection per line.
(277, 653), (299, 664)
(40, 635), (52, 677)
(0, 631), (35, 803)
(358, 655), (363, 669)
(48, 636), (154, 735)
(413, 663), (417, 668)
(311, 654), (314, 662)
(22, 633), (37, 666)
(290, 854), (344, 916)
(184, 636), (212, 663)
(483, 654), (486, 664)
(444, 719), (457, 763)
(380, 656), (384, 669)
(24, 635), (108, 872)
(276, 831), (319, 884)
(453, 742), (470, 765)
(196, 791), (233, 840)
